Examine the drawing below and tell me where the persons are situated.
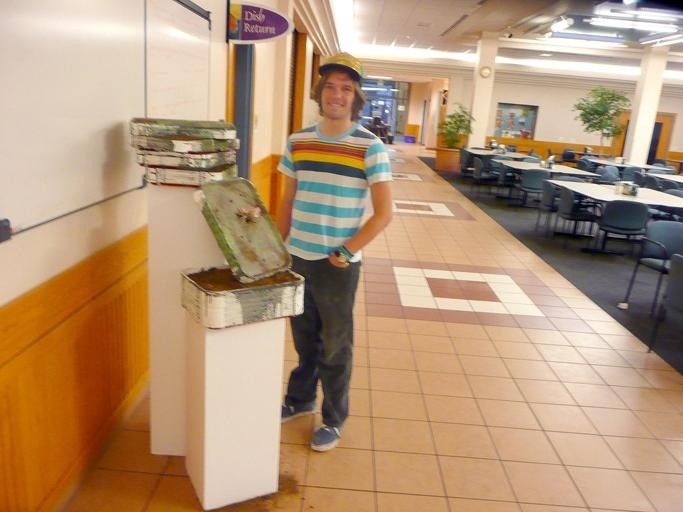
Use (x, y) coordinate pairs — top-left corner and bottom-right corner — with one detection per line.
(277, 53), (391, 452)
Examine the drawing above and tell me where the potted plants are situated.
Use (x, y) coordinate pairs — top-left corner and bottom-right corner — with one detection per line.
(435, 102), (475, 172)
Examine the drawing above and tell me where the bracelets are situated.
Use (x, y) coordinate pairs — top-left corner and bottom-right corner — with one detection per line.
(337, 245), (353, 261)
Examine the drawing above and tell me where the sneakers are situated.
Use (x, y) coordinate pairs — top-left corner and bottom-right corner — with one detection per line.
(281, 399), (316, 423)
(310, 422), (344, 452)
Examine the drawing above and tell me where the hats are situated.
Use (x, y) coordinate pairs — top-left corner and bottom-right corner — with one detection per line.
(319, 52), (363, 81)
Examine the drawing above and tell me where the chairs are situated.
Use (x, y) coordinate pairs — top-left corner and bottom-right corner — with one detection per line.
(647, 253), (683, 356)
(535, 179), (560, 233)
(594, 201), (648, 257)
(551, 189), (600, 249)
(624, 220), (682, 317)
(461, 142), (683, 209)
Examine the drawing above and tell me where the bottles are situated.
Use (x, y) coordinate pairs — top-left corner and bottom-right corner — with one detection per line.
(622, 182), (638, 196)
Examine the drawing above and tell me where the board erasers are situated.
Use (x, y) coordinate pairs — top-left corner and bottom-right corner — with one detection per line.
(0, 219), (11, 243)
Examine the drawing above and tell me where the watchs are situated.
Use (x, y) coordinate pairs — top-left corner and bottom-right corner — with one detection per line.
(334, 249), (351, 266)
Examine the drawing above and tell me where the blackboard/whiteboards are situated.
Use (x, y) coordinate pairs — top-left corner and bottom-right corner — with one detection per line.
(0, 0), (149, 236)
